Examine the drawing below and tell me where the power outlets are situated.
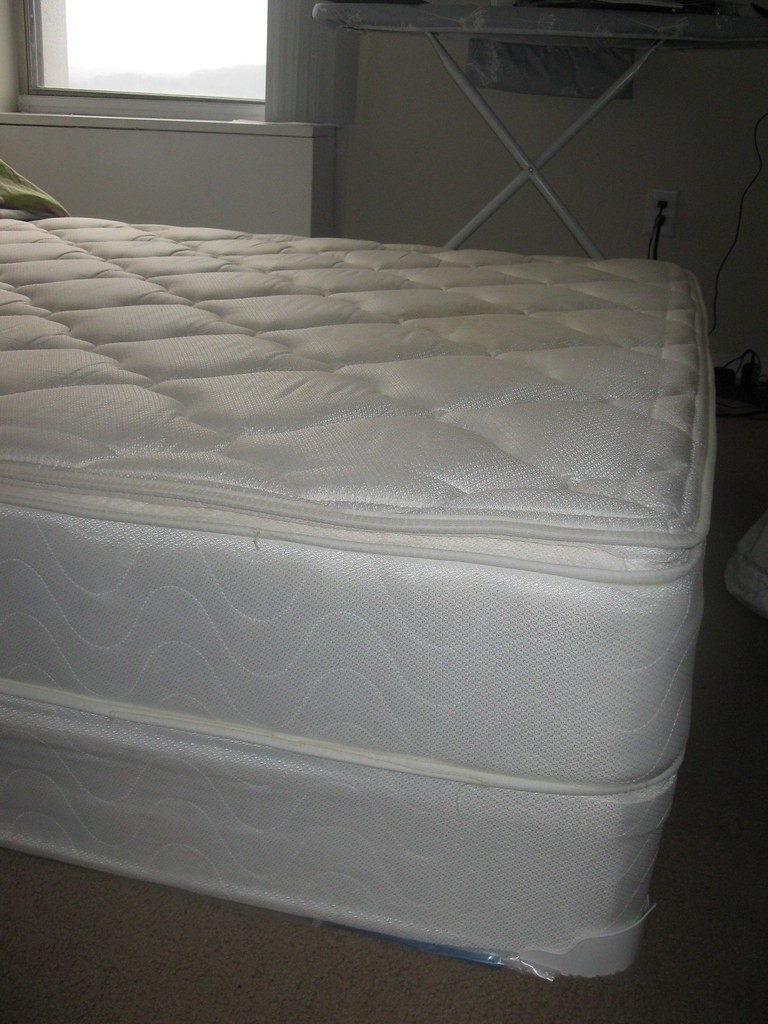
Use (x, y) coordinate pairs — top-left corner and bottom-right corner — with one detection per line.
(642, 189), (679, 239)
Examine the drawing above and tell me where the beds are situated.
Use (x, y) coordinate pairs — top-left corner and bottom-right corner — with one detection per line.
(0, 209), (717, 978)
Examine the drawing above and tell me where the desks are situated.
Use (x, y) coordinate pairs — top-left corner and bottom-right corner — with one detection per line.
(313, 2), (768, 259)
